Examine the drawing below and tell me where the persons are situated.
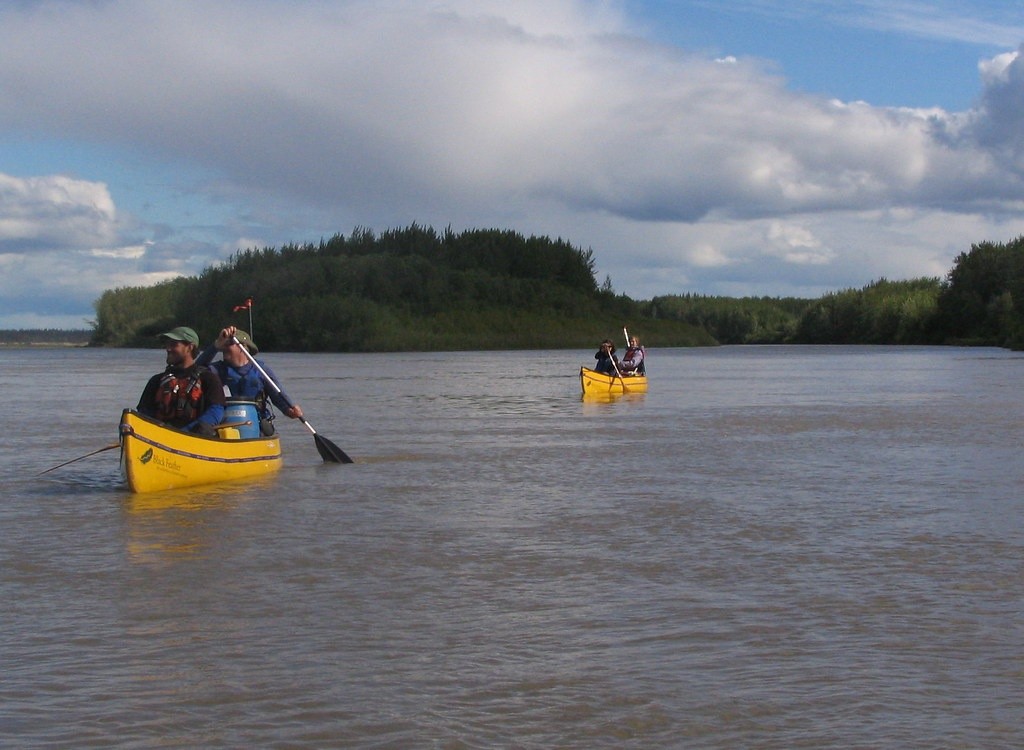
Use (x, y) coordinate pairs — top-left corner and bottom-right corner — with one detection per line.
(593, 338), (618, 377)
(135, 325), (228, 438)
(194, 325), (303, 438)
(615, 323), (645, 377)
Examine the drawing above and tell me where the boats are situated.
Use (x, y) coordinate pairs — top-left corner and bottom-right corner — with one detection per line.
(118, 406), (282, 492)
(579, 365), (646, 396)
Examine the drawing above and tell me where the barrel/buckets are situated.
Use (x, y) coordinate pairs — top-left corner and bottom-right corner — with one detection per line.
(221, 401), (261, 440)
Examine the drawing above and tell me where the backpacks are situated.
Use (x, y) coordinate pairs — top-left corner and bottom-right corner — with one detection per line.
(154, 365), (212, 428)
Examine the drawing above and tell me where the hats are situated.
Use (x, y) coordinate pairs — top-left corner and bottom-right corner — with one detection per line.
(159, 326), (199, 348)
(225, 329), (258, 356)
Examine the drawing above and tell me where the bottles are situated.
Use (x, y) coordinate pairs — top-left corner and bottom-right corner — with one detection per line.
(218, 426), (241, 440)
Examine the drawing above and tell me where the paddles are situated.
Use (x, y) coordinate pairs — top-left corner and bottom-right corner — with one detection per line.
(34, 420), (253, 478)
(606, 345), (633, 396)
(232, 335), (355, 463)
